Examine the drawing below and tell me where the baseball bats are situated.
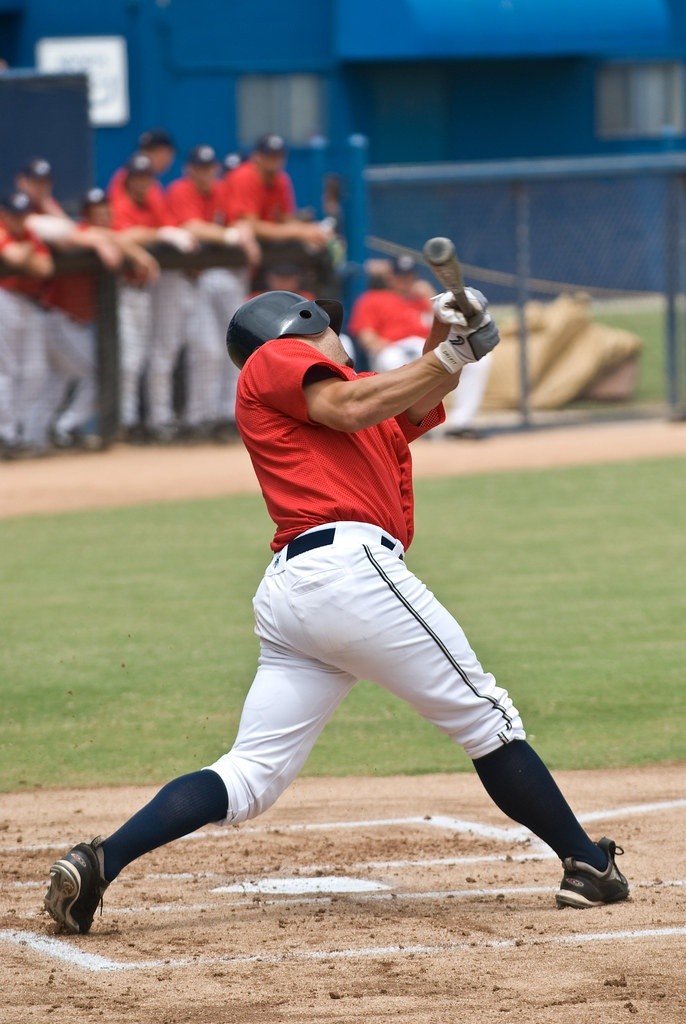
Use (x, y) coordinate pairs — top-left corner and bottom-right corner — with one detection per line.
(424, 236), (478, 326)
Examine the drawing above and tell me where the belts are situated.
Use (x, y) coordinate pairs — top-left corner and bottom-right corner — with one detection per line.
(286, 528), (405, 564)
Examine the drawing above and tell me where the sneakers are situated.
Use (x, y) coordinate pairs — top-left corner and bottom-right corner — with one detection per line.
(44, 835), (109, 935)
(554, 835), (629, 909)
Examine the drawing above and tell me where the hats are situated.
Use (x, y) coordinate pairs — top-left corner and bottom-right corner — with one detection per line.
(223, 152), (247, 171)
(256, 134), (287, 151)
(392, 256), (418, 274)
(3, 192), (32, 212)
(191, 145), (216, 164)
(128, 156), (153, 176)
(25, 159), (52, 179)
(80, 188), (106, 205)
(139, 131), (171, 149)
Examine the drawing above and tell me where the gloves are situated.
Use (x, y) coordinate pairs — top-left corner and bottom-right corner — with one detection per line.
(430, 286), (488, 326)
(432, 316), (502, 374)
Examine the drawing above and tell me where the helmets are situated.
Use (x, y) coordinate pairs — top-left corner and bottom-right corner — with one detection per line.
(226, 290), (344, 371)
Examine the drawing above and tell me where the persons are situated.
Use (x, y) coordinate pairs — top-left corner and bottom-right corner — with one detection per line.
(0, 124), (492, 459)
(45, 283), (628, 937)
